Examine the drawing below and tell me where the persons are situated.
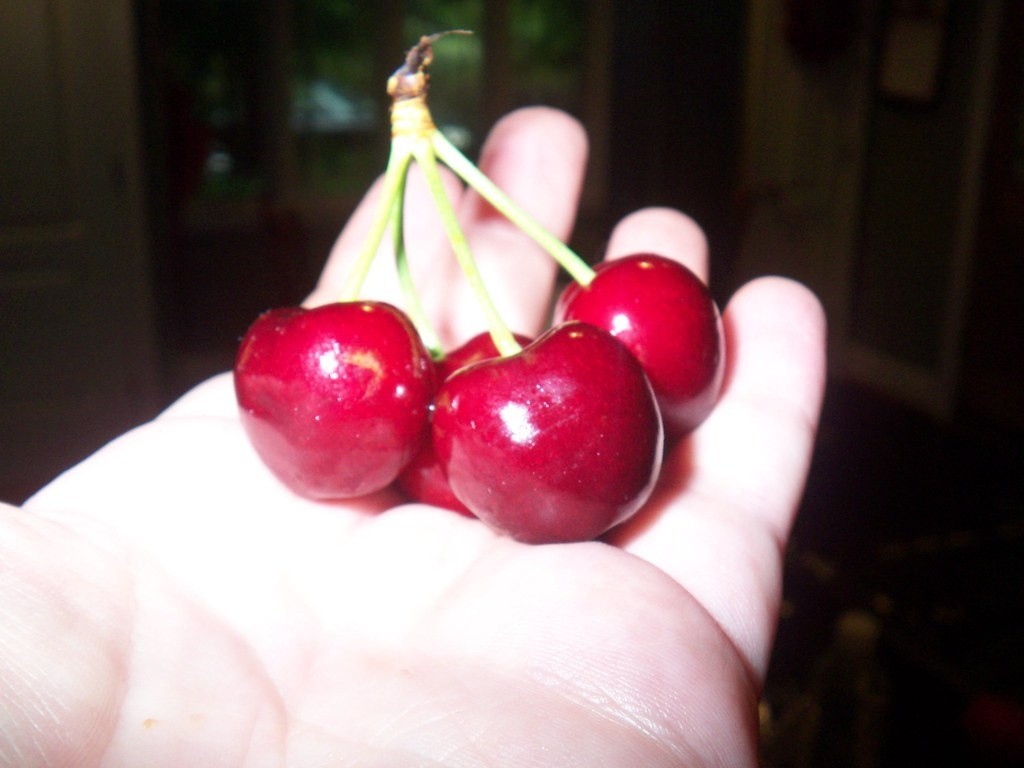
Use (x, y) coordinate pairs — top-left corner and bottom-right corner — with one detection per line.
(1, 106), (829, 768)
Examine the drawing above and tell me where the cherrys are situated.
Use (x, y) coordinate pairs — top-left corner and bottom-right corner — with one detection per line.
(228, 29), (726, 545)
